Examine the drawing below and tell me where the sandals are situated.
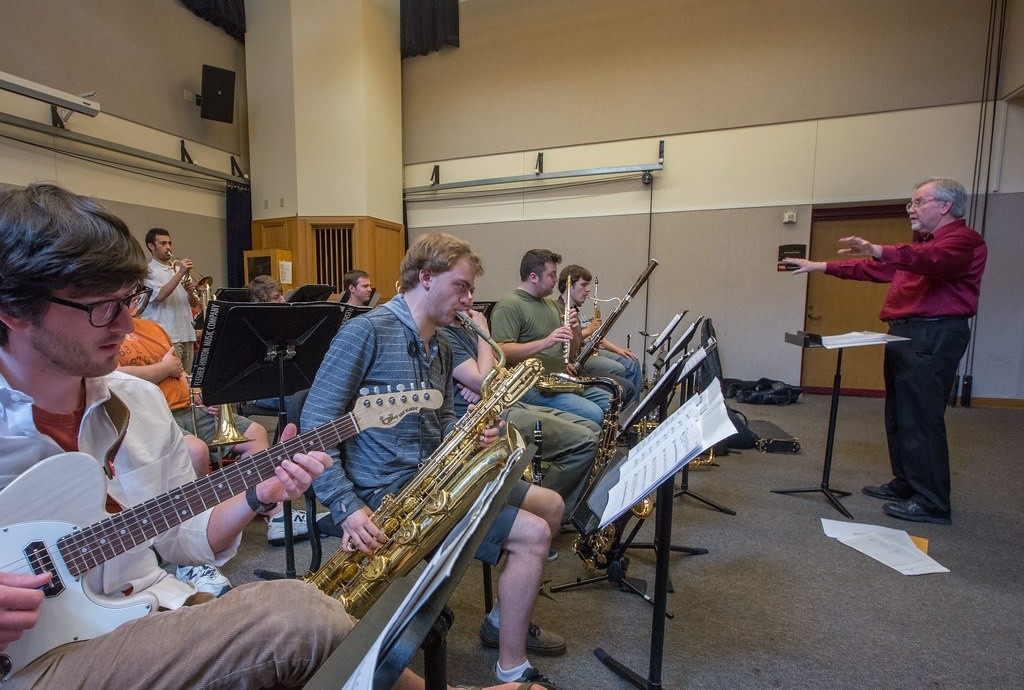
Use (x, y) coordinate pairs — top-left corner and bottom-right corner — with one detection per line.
(456, 683), (559, 690)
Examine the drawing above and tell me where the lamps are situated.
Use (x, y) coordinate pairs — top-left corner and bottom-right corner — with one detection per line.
(0, 70), (100, 118)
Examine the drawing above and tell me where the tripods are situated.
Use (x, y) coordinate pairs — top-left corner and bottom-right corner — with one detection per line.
(549, 336), (738, 619)
(770, 330), (912, 521)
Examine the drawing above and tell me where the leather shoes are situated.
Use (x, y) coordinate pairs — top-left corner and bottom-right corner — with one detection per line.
(862, 478), (918, 501)
(882, 493), (952, 524)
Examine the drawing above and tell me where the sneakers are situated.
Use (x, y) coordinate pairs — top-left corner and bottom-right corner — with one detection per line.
(268, 509), (330, 546)
(488, 665), (559, 690)
(480, 614), (566, 655)
(176, 563), (233, 598)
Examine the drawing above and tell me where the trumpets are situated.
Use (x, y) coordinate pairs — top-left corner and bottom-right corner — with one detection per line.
(166, 250), (214, 300)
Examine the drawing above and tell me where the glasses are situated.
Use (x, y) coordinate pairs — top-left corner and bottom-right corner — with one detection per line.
(906, 199), (944, 211)
(46, 285), (154, 327)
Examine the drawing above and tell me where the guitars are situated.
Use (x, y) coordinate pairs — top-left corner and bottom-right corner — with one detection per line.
(0, 380), (446, 686)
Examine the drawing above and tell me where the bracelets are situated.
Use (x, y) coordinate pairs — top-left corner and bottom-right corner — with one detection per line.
(246, 485), (282, 514)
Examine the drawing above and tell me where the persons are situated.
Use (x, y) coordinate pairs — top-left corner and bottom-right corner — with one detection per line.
(439, 283), (602, 559)
(556, 265), (640, 403)
(118, 287), (339, 545)
(301, 234), (563, 690)
(248, 276), (292, 410)
(136, 228), (198, 376)
(1, 181), (550, 690)
(340, 271), (372, 309)
(491, 247), (614, 425)
(783, 178), (988, 522)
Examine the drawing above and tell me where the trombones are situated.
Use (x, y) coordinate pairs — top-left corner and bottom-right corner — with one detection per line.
(187, 283), (259, 471)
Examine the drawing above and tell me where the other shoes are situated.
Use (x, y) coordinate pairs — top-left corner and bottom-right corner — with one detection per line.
(548, 550), (559, 560)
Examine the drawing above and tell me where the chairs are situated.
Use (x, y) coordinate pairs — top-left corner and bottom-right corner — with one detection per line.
(287, 388), (494, 614)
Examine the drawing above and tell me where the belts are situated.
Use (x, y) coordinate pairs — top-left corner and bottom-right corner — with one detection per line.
(888, 316), (963, 326)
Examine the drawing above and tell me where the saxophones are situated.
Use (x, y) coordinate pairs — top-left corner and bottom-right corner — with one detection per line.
(593, 276), (605, 356)
(549, 371), (654, 577)
(535, 374), (591, 397)
(297, 309), (547, 620)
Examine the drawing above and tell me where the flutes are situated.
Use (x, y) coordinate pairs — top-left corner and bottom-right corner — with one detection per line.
(563, 274), (572, 365)
(530, 419), (543, 486)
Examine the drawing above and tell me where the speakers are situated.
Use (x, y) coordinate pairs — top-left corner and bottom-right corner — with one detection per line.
(200, 63), (236, 123)
